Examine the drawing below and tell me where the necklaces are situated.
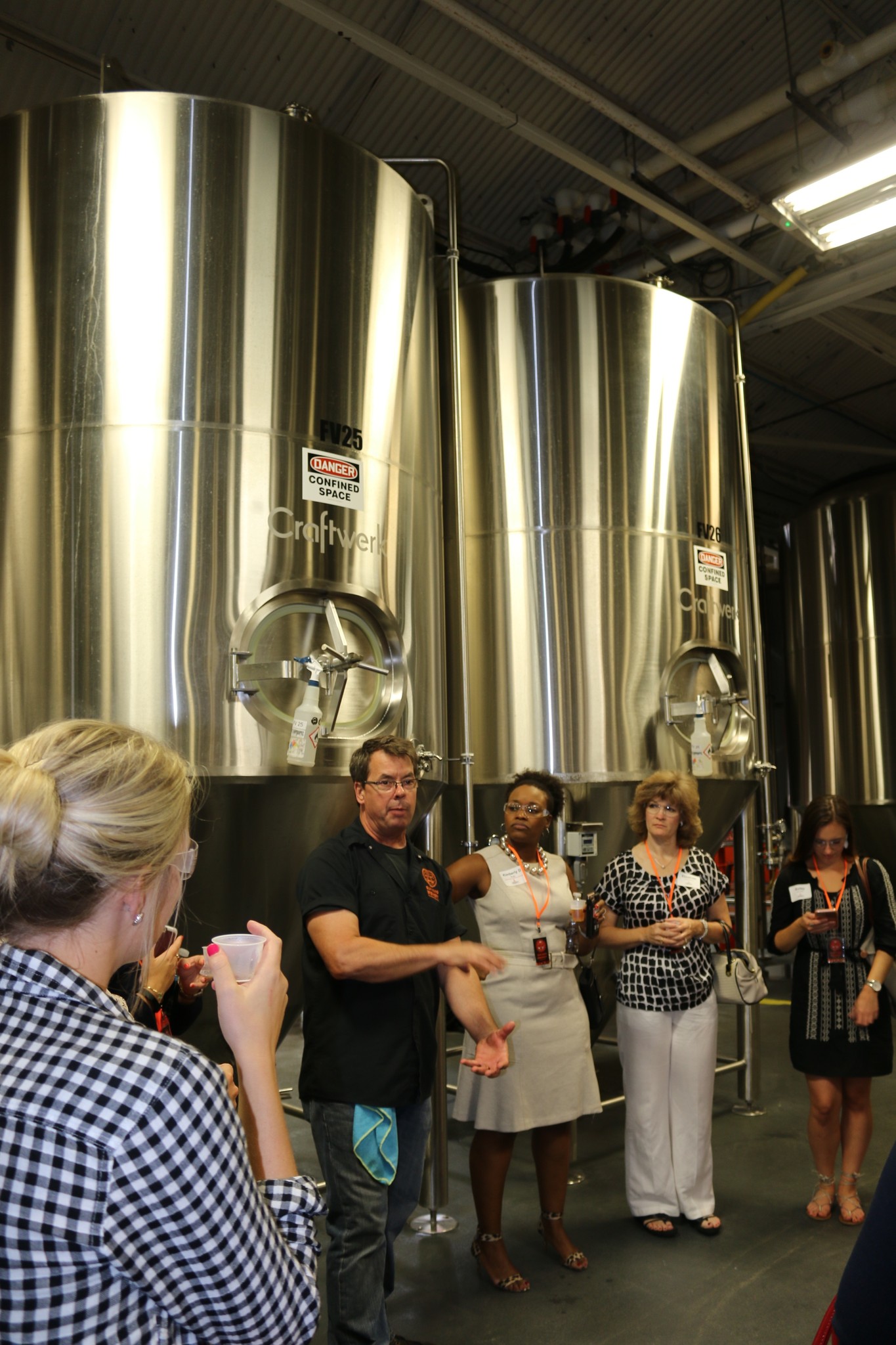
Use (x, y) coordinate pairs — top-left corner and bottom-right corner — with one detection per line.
(649, 843), (679, 868)
(500, 833), (548, 875)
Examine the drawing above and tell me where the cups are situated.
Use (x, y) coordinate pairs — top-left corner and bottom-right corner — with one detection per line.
(569, 900), (586, 922)
(211, 934), (267, 983)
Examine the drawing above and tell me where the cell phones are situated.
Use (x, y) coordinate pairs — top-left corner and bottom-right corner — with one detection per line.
(815, 908), (839, 930)
(153, 925), (178, 958)
(587, 892), (599, 939)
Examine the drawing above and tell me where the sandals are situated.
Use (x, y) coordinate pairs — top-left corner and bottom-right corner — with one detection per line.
(836, 1173), (864, 1226)
(635, 1212), (678, 1238)
(806, 1176), (837, 1221)
(686, 1211), (720, 1236)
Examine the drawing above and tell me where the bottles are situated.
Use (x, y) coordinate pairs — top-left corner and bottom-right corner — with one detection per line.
(564, 891), (583, 954)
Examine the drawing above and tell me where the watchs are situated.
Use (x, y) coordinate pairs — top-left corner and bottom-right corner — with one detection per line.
(177, 976), (203, 999)
(138, 988), (163, 1012)
(865, 979), (882, 992)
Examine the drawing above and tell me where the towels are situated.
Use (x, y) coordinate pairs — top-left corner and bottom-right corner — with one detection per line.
(352, 1104), (399, 1186)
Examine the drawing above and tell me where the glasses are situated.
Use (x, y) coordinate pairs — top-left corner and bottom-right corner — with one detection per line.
(360, 779), (418, 791)
(158, 837), (198, 881)
(646, 803), (683, 817)
(504, 802), (550, 818)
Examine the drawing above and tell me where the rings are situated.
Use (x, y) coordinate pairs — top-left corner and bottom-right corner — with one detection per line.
(603, 915), (605, 920)
(659, 937), (663, 944)
(176, 954), (179, 958)
(683, 938), (687, 944)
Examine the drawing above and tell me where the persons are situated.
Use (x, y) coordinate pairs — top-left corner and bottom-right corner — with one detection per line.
(592, 769), (732, 1238)
(0, 717), (327, 1345)
(108, 934), (213, 1032)
(766, 795), (896, 1225)
(832, 1141), (896, 1345)
(445, 767), (606, 1294)
(297, 734), (514, 1345)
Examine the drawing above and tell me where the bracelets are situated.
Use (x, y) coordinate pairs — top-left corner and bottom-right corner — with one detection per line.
(143, 985), (163, 1001)
(578, 926), (589, 938)
(694, 919), (708, 940)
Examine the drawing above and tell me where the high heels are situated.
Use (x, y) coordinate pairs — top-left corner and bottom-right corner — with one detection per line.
(539, 1212), (588, 1271)
(470, 1221), (529, 1293)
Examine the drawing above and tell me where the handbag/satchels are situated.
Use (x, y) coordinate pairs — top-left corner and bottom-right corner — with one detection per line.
(707, 918), (769, 1006)
(855, 856), (896, 997)
(571, 932), (601, 1014)
